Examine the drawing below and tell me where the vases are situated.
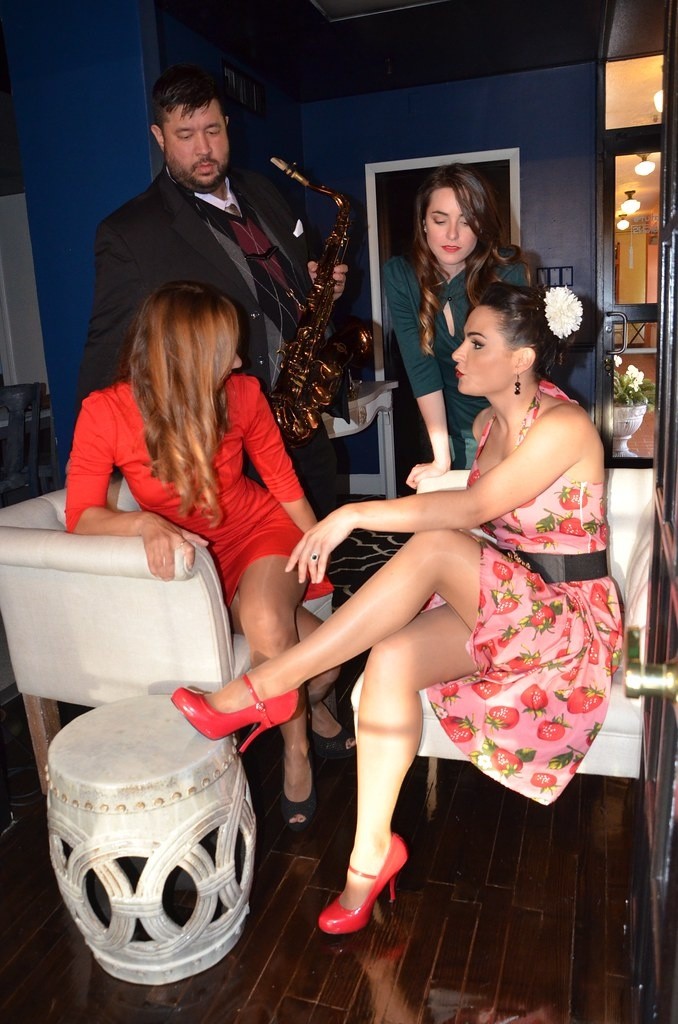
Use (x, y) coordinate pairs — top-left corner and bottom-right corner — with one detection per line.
(613, 405), (647, 437)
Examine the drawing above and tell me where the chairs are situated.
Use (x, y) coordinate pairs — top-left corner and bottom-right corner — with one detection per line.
(352, 469), (656, 778)
(0, 476), (333, 795)
(0, 381), (43, 509)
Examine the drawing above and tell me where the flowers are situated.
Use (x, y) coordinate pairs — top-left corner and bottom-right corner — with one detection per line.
(614, 355), (656, 413)
(543, 284), (583, 340)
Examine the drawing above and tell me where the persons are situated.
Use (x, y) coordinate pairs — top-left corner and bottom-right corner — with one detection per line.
(382, 163), (532, 490)
(75, 62), (349, 525)
(65, 284), (356, 834)
(172, 287), (627, 934)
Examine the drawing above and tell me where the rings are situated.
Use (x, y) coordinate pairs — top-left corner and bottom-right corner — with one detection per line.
(310, 554), (319, 560)
(179, 539), (189, 547)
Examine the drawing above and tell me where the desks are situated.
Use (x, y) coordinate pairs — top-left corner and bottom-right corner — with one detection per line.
(0, 406), (54, 507)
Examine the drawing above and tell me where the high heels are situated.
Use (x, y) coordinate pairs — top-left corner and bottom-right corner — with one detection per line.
(317, 831), (409, 935)
(280, 737), (317, 831)
(306, 701), (355, 759)
(171, 673), (299, 757)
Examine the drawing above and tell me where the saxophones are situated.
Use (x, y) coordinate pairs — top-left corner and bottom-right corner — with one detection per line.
(266, 153), (372, 449)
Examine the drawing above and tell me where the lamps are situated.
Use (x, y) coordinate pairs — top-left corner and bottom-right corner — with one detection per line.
(621, 190), (641, 215)
(634, 152), (654, 177)
(617, 215), (629, 231)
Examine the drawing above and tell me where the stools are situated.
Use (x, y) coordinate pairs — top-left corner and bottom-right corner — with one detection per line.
(44, 695), (256, 986)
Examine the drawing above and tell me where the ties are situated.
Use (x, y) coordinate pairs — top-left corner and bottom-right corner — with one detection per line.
(225, 204), (241, 218)
